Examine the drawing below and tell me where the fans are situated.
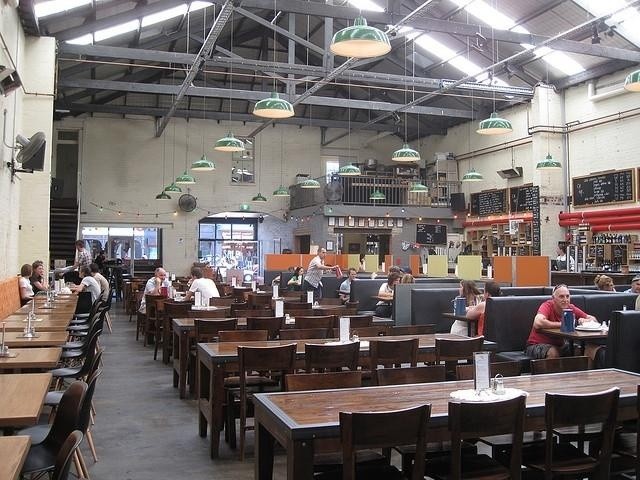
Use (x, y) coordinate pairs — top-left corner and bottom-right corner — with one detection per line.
(14, 130), (47, 163)
(178, 193), (199, 213)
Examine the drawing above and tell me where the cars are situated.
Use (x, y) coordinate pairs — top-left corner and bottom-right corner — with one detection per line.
(243, 263), (258, 282)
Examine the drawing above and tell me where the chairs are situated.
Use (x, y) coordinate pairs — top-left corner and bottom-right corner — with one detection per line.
(114, 270), (639, 479)
(0, 269), (112, 480)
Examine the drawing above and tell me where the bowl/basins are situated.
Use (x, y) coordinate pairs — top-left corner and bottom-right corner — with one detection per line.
(582, 321), (601, 328)
(578, 332), (600, 336)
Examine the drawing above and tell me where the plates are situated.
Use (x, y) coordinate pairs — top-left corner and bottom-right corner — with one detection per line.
(574, 326), (601, 332)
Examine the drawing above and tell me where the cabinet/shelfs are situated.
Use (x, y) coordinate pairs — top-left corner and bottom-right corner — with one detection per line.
(460, 218), (534, 258)
(582, 233), (639, 269)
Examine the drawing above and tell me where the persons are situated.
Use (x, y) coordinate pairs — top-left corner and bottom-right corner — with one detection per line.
(624, 274), (640, 293)
(449, 279), (482, 336)
(272, 238), (416, 320)
(593, 273), (617, 292)
(523, 283), (599, 359)
(186, 266), (221, 307)
(555, 241), (577, 272)
(584, 295), (640, 370)
(138, 267), (167, 346)
(17, 235), (135, 307)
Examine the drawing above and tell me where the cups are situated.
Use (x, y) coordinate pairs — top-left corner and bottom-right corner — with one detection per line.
(629, 264), (640, 271)
(628, 250), (640, 258)
(175, 293), (181, 300)
(60, 287), (70, 292)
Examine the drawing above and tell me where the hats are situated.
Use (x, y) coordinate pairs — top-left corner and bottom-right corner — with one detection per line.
(399, 266), (411, 273)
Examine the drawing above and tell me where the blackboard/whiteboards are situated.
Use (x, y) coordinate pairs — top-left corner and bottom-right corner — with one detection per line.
(415, 222), (447, 247)
(572, 168), (635, 208)
(635, 167), (640, 204)
(477, 186), (540, 249)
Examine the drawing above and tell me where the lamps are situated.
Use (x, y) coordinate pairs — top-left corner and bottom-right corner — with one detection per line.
(408, 113), (429, 193)
(337, 57), (362, 176)
(190, 66), (215, 171)
(272, 123), (290, 196)
(9, 137), (46, 184)
(252, 0), (296, 118)
(369, 130), (386, 200)
(0, 64), (22, 98)
(300, 101), (321, 189)
(461, 89), (484, 183)
(476, 2), (513, 137)
(155, 132), (172, 200)
(623, 68), (640, 95)
(329, 2), (392, 60)
(252, 132), (267, 202)
(164, 118), (182, 193)
(536, 46), (562, 171)
(391, 23), (421, 162)
(176, 96), (196, 185)
(214, 2), (246, 153)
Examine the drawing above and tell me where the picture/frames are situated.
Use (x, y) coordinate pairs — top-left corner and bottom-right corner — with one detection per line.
(328, 216), (404, 228)
(326, 240), (335, 252)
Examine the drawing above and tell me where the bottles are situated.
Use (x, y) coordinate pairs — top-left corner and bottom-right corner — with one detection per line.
(598, 260), (622, 270)
(494, 380), (505, 396)
(592, 233), (631, 244)
(601, 321), (608, 332)
(352, 334), (360, 344)
(285, 313), (290, 324)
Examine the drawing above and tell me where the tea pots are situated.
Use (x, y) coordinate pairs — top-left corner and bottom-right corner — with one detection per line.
(335, 266), (344, 279)
(451, 296), (466, 315)
(560, 308), (574, 332)
(157, 287), (169, 298)
